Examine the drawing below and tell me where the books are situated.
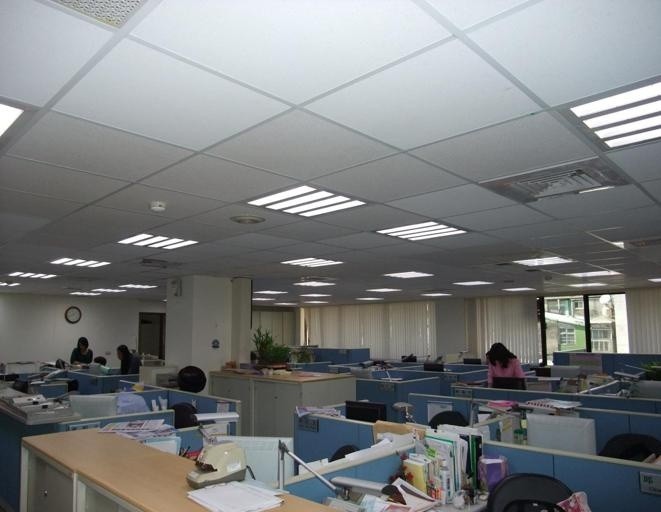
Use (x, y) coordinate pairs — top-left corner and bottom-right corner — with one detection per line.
(403, 434), (482, 505)
(296, 406), (339, 418)
(186, 480), (285, 512)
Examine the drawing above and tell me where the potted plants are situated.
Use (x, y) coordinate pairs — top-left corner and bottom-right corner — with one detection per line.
(252, 327), (290, 370)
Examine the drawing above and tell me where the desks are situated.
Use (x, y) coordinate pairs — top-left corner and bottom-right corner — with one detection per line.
(0, 348), (661, 512)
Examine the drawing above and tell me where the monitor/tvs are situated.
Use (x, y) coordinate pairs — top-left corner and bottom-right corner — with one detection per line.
(530, 367), (551, 377)
(69, 395), (116, 417)
(401, 356), (416, 362)
(463, 358), (481, 365)
(423, 363), (443, 372)
(13, 378), (29, 393)
(492, 376), (527, 390)
(88, 363), (102, 375)
(345, 400), (387, 423)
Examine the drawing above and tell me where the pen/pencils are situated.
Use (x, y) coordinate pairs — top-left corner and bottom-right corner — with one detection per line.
(178, 446), (191, 459)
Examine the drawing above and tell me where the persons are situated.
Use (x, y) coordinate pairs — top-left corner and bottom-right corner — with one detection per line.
(70, 337), (93, 364)
(117, 345), (139, 374)
(487, 343), (525, 388)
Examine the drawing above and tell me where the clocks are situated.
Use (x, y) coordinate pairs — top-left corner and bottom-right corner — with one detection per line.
(64, 306), (82, 323)
